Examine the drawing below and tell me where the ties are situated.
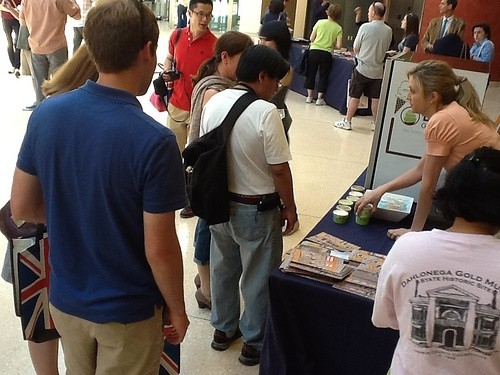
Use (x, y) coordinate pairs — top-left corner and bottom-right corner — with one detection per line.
(439, 20), (448, 39)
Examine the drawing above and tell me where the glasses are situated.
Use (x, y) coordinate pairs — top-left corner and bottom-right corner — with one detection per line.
(258, 37), (273, 44)
(191, 9), (213, 18)
(267, 74), (284, 88)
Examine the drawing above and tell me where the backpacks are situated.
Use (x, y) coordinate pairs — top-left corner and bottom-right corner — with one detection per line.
(182, 91), (261, 226)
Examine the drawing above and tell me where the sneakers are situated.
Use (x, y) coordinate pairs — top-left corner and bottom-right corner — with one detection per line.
(335, 119), (352, 131)
(370, 121), (375, 131)
(211, 319), (242, 351)
(239, 344), (262, 366)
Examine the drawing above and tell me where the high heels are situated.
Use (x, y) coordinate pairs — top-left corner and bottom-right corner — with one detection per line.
(195, 288), (212, 310)
(194, 274), (201, 291)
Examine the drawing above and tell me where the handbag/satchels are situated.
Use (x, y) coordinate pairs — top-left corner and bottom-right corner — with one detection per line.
(0, 201), (47, 244)
(9, 224), (63, 344)
(159, 310), (181, 375)
(150, 91), (169, 112)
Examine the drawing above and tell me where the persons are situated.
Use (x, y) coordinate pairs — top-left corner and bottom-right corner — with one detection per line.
(370, 145), (500, 375)
(9, 0), (191, 374)
(333, 1), (393, 131)
(177, 0), (188, 28)
(161, 0), (218, 217)
(421, 0), (465, 54)
(257, 20), (294, 134)
(386, 11), (420, 60)
(198, 43), (298, 365)
(0, 0), (92, 111)
(312, 0), (330, 29)
(468, 23), (495, 64)
(2, 43), (100, 375)
(353, 58), (500, 241)
(185, 29), (259, 310)
(259, 0), (288, 25)
(304, 3), (343, 106)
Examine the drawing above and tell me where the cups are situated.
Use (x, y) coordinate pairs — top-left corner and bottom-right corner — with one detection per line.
(358, 204), (373, 220)
(333, 210), (349, 224)
(335, 205), (352, 215)
(338, 185), (366, 208)
(355, 212), (369, 225)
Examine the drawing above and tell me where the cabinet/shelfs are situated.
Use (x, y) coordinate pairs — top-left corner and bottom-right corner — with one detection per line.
(365, 50), (491, 204)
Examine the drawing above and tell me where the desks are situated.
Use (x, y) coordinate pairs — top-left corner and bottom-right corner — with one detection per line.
(259, 168), (451, 375)
(288, 41), (373, 117)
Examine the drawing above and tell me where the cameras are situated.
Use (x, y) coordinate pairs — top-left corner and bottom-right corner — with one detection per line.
(165, 69), (180, 80)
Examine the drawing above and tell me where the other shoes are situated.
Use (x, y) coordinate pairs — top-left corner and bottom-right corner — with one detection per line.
(9, 66), (20, 77)
(305, 96), (328, 106)
(23, 102), (36, 111)
